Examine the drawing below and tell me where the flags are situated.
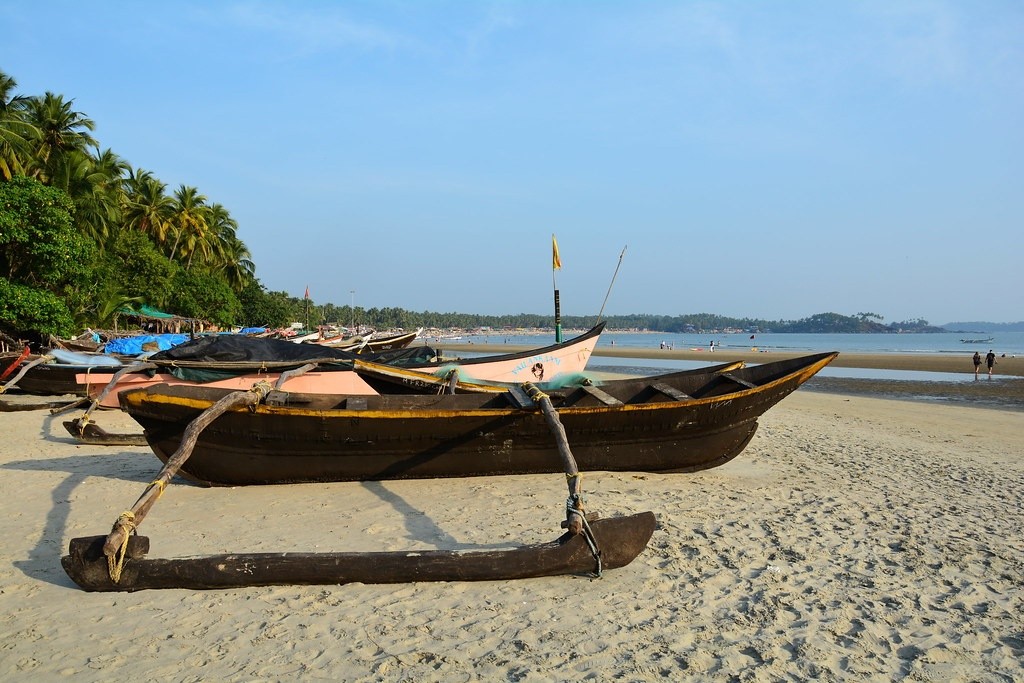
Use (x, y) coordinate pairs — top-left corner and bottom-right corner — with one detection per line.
(551, 234), (561, 270)
(304, 288), (309, 298)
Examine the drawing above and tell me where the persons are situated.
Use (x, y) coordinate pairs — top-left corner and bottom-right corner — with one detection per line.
(424, 339), (428, 346)
(710, 341), (714, 352)
(972, 352), (982, 373)
(985, 349), (998, 374)
(662, 340), (665, 349)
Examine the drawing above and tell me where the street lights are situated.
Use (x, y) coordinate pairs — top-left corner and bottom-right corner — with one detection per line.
(350, 290), (355, 328)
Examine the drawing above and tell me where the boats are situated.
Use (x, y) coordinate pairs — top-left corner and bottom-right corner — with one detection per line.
(0, 323), (839, 595)
